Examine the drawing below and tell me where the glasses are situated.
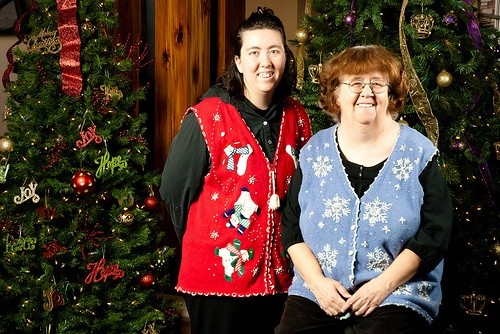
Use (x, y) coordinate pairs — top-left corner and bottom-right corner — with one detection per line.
(340, 79), (390, 93)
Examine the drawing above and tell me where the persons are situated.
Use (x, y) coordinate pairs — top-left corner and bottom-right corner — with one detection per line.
(159, 7), (312, 334)
(276, 45), (452, 334)
